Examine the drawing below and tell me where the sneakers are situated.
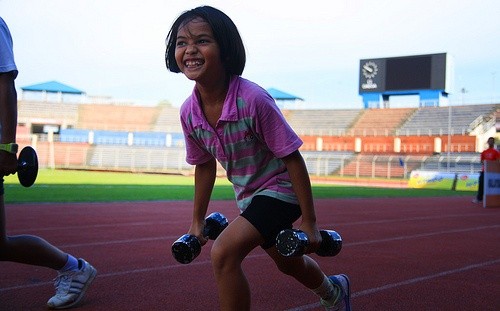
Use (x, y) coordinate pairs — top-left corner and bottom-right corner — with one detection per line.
(321, 274), (351, 311)
(47, 258), (98, 309)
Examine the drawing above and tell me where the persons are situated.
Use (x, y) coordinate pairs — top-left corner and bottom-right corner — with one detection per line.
(473, 137), (500, 203)
(0, 16), (98, 308)
(165, 5), (353, 311)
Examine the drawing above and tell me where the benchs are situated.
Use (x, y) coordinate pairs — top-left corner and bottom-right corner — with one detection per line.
(14, 99), (500, 178)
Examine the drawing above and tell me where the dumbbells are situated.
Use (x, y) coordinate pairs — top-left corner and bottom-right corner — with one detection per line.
(170, 212), (230, 264)
(274, 227), (343, 260)
(0, 143), (38, 188)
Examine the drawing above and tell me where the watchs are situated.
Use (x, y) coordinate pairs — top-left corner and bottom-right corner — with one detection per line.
(0, 143), (18, 154)
